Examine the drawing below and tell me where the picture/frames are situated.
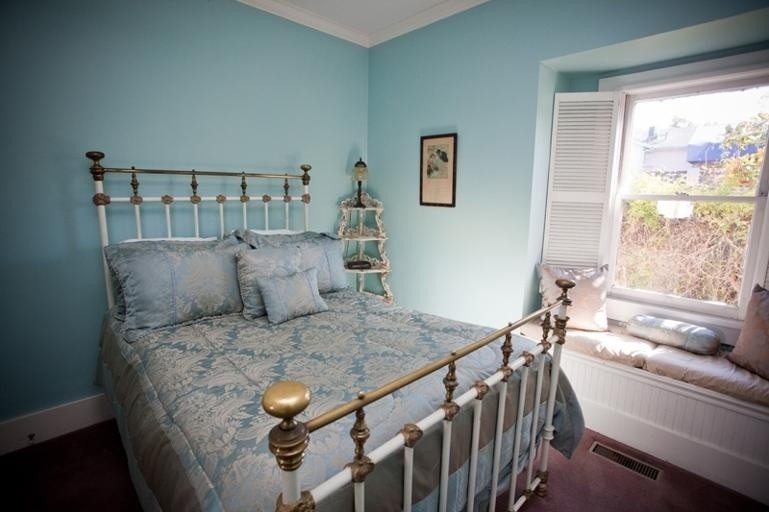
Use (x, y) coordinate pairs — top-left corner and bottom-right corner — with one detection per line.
(420, 133), (457, 207)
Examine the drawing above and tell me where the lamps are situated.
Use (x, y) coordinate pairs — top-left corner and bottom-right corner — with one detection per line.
(351, 157), (369, 208)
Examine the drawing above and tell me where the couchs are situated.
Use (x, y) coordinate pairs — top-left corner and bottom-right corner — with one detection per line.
(524, 318), (769, 503)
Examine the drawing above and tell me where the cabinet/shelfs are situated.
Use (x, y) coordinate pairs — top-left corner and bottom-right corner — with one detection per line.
(340, 191), (394, 304)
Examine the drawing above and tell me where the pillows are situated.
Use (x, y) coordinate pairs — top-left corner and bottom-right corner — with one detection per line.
(234, 228), (317, 243)
(257, 236), (347, 292)
(618, 314), (728, 355)
(102, 233), (238, 321)
(236, 246), (302, 320)
(104, 241), (253, 343)
(536, 263), (609, 331)
(255, 267), (328, 326)
(725, 284), (769, 382)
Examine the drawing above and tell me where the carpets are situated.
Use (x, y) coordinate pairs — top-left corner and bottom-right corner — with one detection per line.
(495, 427), (769, 512)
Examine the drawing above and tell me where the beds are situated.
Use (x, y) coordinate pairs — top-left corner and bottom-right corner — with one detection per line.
(85, 151), (576, 512)
